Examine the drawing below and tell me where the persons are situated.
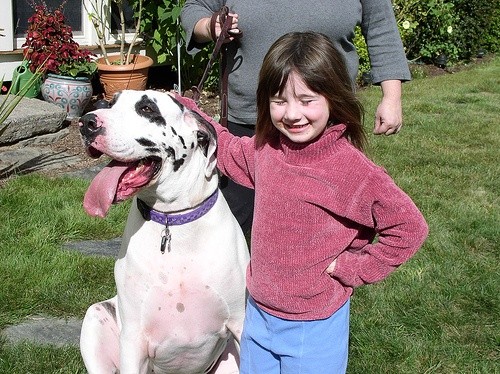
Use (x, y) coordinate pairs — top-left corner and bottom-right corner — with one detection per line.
(146, 30), (429, 373)
(179, 0), (411, 259)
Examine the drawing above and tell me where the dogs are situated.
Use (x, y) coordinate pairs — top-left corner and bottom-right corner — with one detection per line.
(79, 89), (250, 374)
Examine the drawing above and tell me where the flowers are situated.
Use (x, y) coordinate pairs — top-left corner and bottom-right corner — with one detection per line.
(1, 0), (97, 125)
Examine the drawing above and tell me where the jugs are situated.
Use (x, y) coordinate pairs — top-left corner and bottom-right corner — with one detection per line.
(9, 56), (42, 98)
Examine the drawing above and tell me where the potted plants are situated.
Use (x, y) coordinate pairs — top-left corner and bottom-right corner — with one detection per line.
(41, 58), (97, 122)
(84, 0), (153, 103)
